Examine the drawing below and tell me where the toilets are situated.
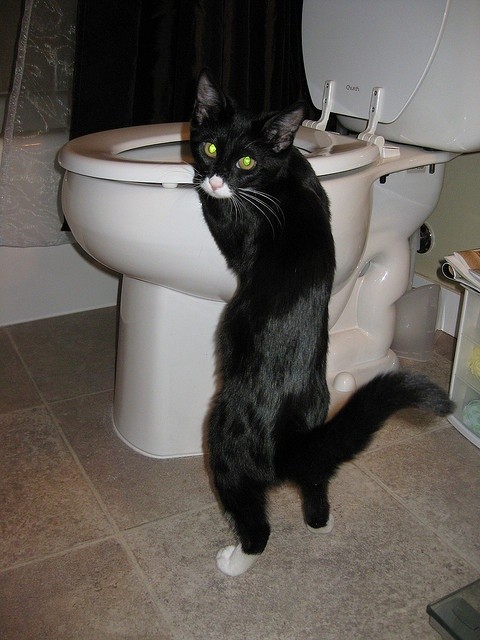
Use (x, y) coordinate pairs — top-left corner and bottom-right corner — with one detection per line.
(55, 1), (477, 462)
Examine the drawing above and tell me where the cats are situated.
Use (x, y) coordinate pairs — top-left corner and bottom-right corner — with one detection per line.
(182, 68), (459, 577)
